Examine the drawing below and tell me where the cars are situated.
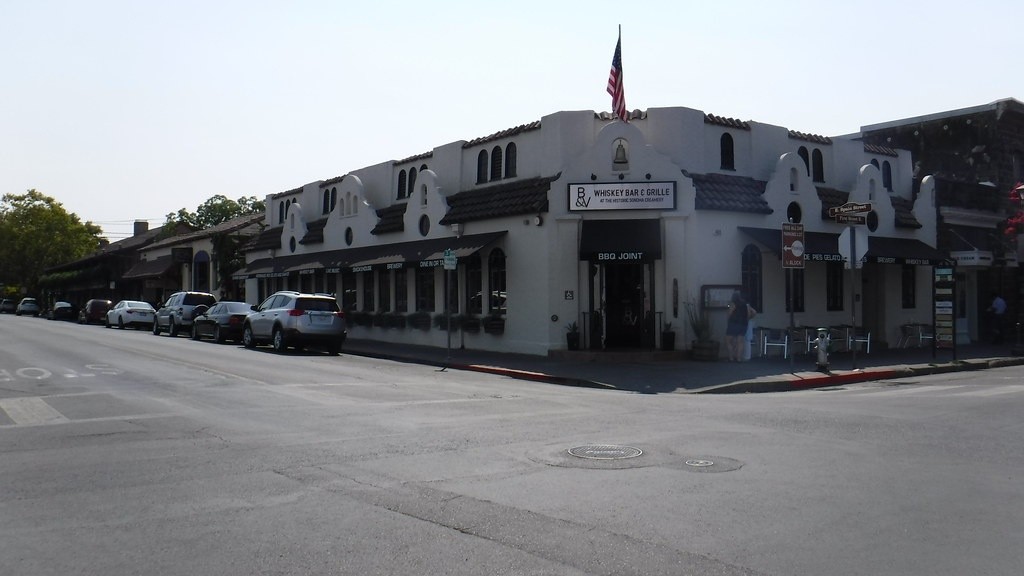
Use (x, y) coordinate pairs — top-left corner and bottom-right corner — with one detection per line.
(47, 301), (76, 322)
(191, 301), (256, 344)
(16, 297), (42, 317)
(0, 298), (18, 314)
(78, 299), (114, 325)
(329, 285), (508, 322)
(104, 300), (157, 331)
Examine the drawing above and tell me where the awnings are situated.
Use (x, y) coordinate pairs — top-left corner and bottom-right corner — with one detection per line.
(122, 258), (180, 280)
(736, 226), (957, 268)
(232, 230), (509, 282)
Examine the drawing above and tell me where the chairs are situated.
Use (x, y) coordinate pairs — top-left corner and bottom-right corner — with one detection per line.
(751, 325), (870, 358)
(896, 323), (934, 350)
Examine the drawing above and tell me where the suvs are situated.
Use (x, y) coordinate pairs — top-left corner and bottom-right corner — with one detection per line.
(153, 291), (219, 338)
(242, 291), (348, 355)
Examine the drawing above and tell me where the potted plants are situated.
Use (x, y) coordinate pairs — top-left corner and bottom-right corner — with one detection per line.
(566, 322), (580, 350)
(662, 322), (675, 351)
(682, 300), (722, 361)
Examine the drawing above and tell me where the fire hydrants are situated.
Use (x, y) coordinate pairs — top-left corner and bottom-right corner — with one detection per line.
(814, 326), (833, 374)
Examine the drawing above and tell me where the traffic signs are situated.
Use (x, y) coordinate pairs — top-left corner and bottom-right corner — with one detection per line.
(781, 221), (805, 269)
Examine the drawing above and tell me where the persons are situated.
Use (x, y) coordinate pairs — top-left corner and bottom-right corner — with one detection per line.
(153, 296), (162, 309)
(988, 293), (1008, 346)
(726, 292), (757, 362)
(219, 291), (229, 302)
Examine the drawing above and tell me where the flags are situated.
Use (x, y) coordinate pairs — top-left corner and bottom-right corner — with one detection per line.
(607, 37), (630, 125)
